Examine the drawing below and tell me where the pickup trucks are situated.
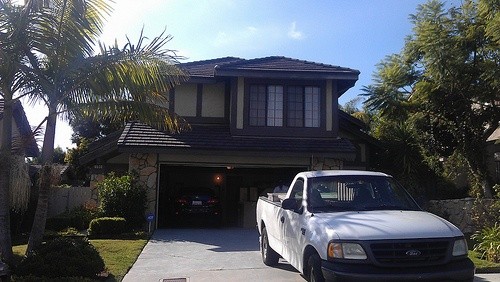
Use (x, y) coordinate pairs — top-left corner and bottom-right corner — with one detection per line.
(256, 170), (475, 282)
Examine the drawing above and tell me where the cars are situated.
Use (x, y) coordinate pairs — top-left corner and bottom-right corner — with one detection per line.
(173, 193), (224, 225)
